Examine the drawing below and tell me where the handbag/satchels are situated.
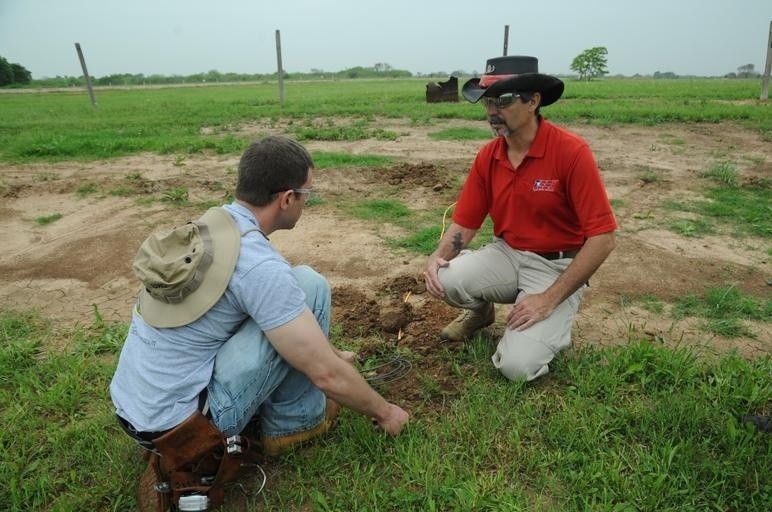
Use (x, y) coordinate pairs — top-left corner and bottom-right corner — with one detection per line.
(152, 409), (259, 510)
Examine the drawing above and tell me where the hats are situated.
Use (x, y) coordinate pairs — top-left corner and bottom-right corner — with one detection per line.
(135, 206), (241, 330)
(463, 56), (564, 108)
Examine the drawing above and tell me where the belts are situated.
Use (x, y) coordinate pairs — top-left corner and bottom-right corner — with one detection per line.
(543, 252), (576, 260)
(115, 415), (159, 443)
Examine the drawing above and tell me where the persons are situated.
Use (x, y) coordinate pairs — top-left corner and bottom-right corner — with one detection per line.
(423, 55), (616, 386)
(107, 137), (410, 512)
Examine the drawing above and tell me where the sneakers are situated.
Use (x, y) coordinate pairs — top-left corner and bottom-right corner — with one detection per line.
(441, 304), (496, 341)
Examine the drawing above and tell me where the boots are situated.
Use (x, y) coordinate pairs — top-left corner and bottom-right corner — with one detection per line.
(261, 397), (341, 455)
(139, 449), (171, 512)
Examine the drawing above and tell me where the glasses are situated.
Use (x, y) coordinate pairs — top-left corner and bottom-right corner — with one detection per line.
(477, 93), (523, 108)
(274, 187), (314, 200)
